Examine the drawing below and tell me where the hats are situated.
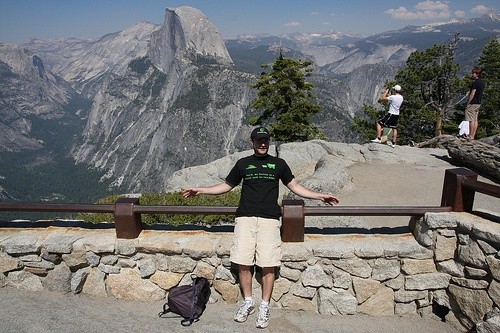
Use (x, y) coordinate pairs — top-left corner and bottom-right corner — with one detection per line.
(251, 126), (270, 140)
(392, 85), (401, 91)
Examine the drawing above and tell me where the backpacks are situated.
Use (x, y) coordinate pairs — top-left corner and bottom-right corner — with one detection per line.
(158, 274), (209, 326)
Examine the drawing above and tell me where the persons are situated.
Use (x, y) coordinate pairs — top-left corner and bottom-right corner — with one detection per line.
(465, 68), (484, 139)
(181, 126), (339, 329)
(370, 84), (403, 147)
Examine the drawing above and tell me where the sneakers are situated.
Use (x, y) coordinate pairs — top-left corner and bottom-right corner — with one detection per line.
(234, 302), (256, 321)
(256, 305), (269, 328)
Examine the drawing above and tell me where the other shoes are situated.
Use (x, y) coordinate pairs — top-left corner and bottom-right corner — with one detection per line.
(371, 137), (381, 143)
(388, 142), (395, 148)
(465, 135), (473, 141)
